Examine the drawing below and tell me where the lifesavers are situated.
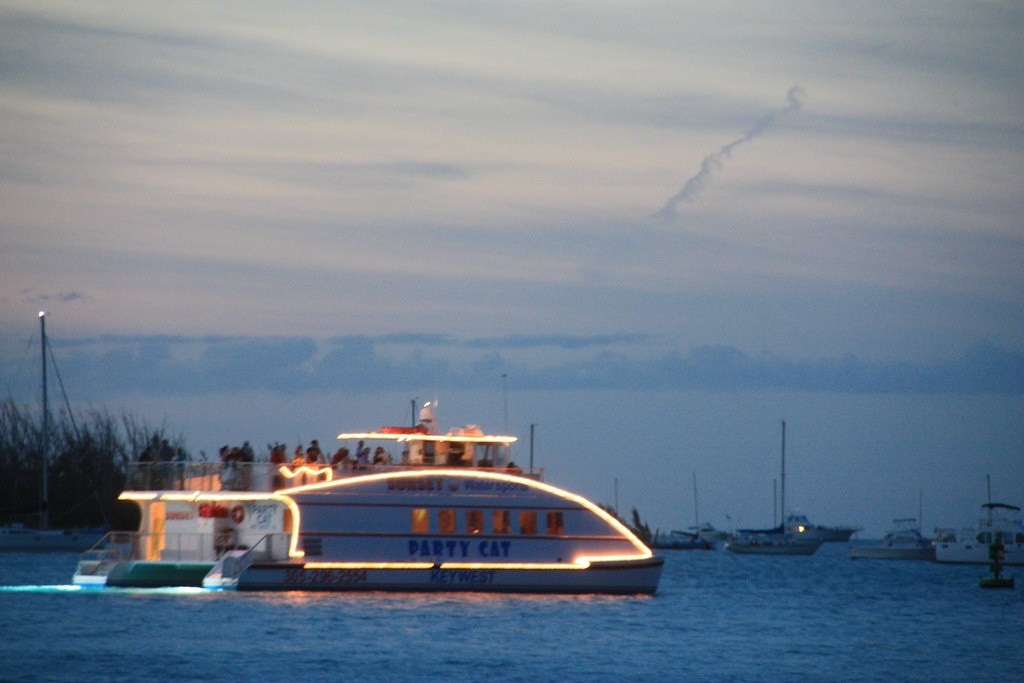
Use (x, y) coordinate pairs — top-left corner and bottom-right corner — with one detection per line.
(231, 505), (244, 523)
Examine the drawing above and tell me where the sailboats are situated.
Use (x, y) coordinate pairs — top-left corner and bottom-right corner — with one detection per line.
(0, 312), (106, 550)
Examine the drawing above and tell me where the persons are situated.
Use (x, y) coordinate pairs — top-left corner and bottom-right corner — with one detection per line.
(213, 526), (235, 561)
(219, 441), (255, 492)
(269, 439), (327, 490)
(133, 434), (186, 490)
(332, 440), (391, 472)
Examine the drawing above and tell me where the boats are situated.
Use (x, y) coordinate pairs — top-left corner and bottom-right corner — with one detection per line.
(933, 472), (1024, 564)
(849, 486), (936, 561)
(979, 531), (1016, 588)
(655, 419), (864, 555)
(72, 387), (666, 595)
